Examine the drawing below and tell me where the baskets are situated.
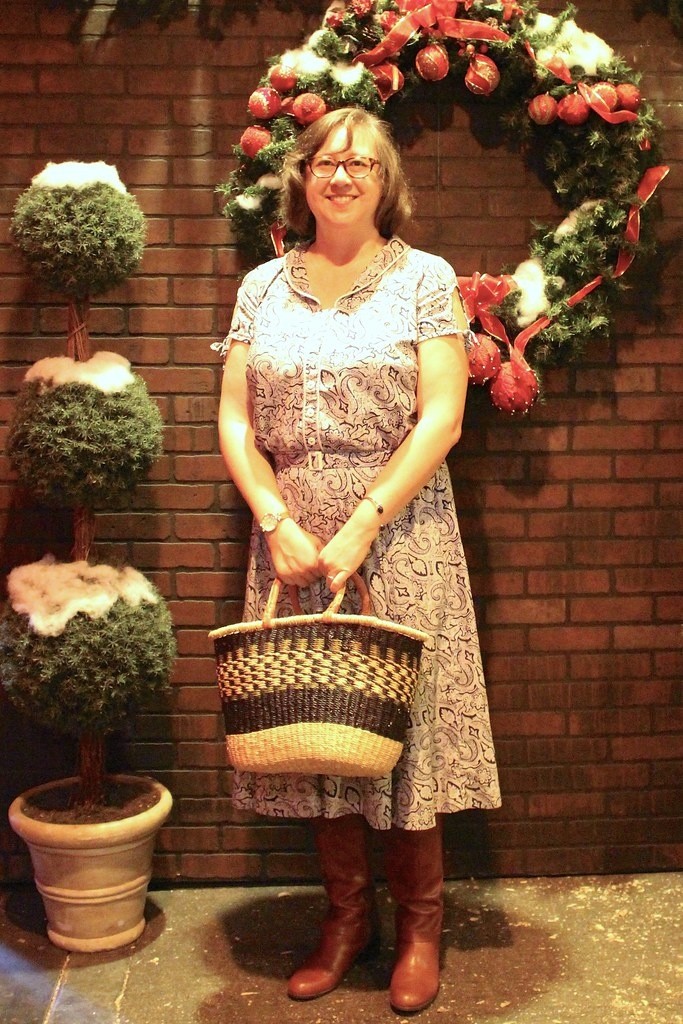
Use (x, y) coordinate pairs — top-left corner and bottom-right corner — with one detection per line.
(207, 571), (429, 778)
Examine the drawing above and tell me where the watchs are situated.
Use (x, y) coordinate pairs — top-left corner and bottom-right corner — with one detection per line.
(258, 511), (291, 534)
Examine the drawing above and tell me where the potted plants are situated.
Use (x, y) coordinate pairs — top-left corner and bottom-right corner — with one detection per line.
(0, 160), (172, 952)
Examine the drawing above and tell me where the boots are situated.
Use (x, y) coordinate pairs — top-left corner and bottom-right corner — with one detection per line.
(382, 812), (444, 1011)
(285, 814), (381, 999)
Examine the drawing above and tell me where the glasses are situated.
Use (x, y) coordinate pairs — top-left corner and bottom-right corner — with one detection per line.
(305, 154), (381, 178)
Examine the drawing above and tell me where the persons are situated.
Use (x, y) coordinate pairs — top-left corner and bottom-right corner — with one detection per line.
(216, 104), (502, 1015)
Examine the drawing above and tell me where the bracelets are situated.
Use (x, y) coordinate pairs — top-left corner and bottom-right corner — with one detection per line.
(362, 496), (387, 531)
